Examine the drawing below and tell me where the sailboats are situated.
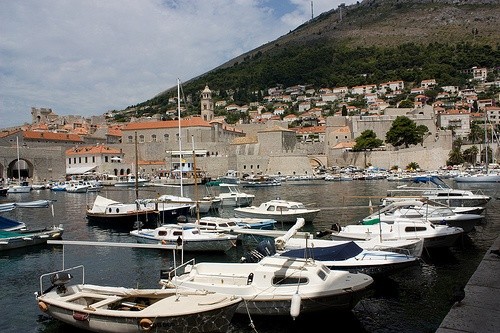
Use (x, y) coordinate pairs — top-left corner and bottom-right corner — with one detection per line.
(84, 130), (191, 226)
(7, 136), (31, 192)
(175, 135), (245, 236)
(129, 133), (237, 251)
(134, 77), (222, 215)
(448, 110), (500, 182)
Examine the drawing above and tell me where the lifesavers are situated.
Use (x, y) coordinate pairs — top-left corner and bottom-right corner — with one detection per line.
(38, 302), (47, 311)
(140, 318), (153, 331)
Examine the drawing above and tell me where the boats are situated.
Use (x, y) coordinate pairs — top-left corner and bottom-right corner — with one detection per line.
(0, 186), (10, 194)
(0, 199), (65, 250)
(148, 159), (210, 186)
(235, 197), (319, 225)
(31, 173), (150, 192)
(197, 214), (278, 230)
(252, 234), (423, 276)
(202, 181), (255, 207)
(158, 238), (374, 314)
(318, 176), (492, 256)
(242, 170), (282, 188)
(33, 244), (244, 333)
(218, 170), (242, 186)
(0, 216), (27, 231)
